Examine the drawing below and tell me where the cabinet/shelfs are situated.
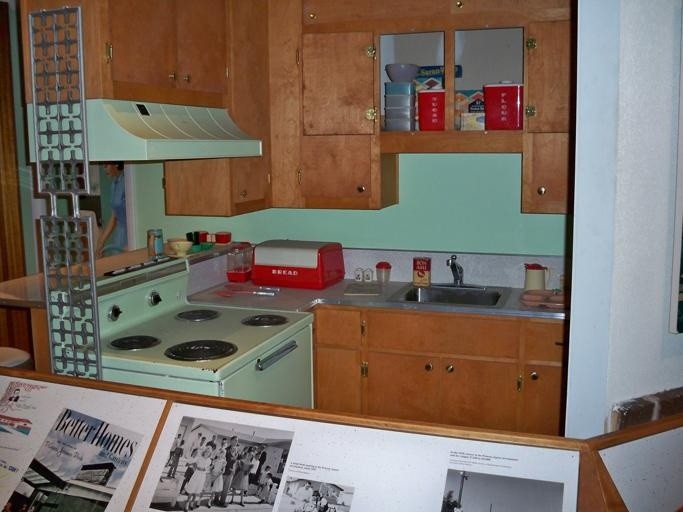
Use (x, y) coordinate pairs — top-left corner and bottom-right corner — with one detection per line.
(20, 1), (230, 109)
(269, 1), (398, 213)
(313, 304), (363, 415)
(520, 1), (571, 216)
(380, 2), (525, 159)
(165, 1), (269, 218)
(522, 317), (564, 440)
(363, 307), (521, 437)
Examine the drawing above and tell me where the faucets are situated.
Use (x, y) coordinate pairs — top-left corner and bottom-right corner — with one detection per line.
(444, 258), (464, 287)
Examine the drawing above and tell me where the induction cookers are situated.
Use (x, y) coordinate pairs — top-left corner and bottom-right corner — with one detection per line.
(52, 271), (316, 380)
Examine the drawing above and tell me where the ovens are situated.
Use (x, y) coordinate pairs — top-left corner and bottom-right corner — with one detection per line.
(220, 324), (314, 409)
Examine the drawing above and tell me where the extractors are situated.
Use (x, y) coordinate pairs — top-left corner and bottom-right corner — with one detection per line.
(27, 98), (262, 164)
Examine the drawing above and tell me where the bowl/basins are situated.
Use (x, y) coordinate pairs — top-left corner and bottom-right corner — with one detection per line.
(168, 241), (192, 255)
(384, 64), (419, 83)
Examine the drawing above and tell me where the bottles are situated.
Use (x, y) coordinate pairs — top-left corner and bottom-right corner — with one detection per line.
(184, 231), (231, 252)
(355, 261), (391, 282)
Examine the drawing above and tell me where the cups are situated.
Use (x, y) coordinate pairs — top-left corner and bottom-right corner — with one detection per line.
(147, 229), (163, 257)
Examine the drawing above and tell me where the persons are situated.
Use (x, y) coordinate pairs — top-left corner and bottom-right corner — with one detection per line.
(165, 431), (274, 512)
(294, 481), (328, 512)
(8, 389), (21, 402)
(95, 164), (128, 258)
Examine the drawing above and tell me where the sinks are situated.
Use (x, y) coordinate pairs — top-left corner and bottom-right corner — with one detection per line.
(384, 282), (516, 309)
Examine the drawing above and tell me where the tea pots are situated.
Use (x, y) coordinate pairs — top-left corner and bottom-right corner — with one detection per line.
(523, 263), (553, 292)
